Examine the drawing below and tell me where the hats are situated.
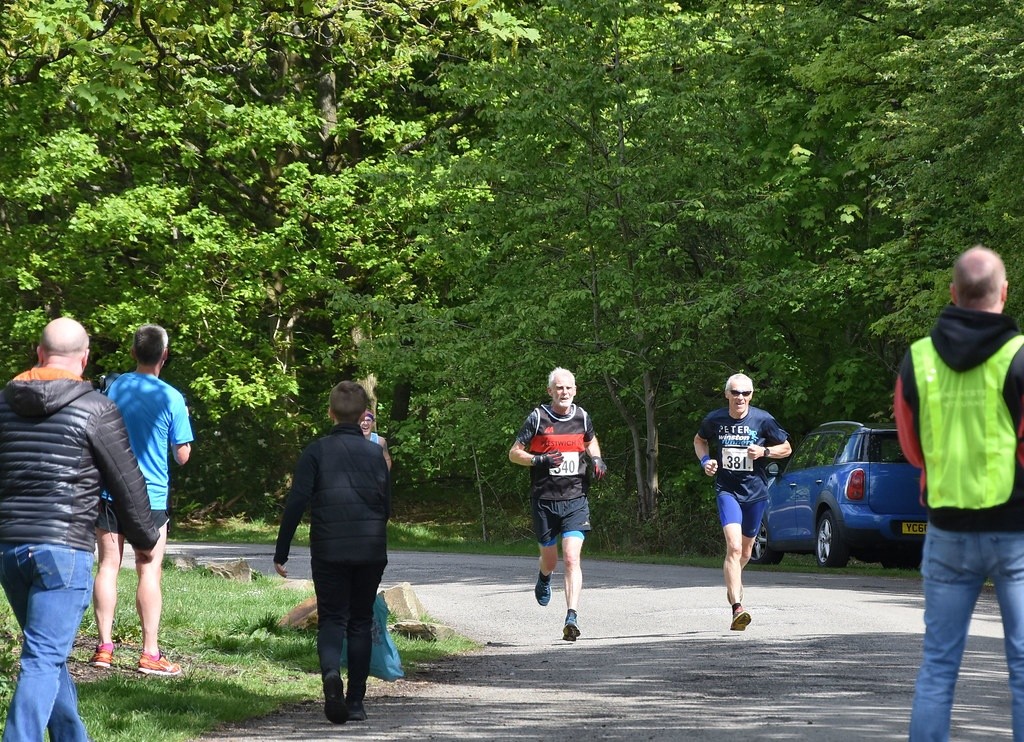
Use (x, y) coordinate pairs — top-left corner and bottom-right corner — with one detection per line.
(365, 411), (375, 421)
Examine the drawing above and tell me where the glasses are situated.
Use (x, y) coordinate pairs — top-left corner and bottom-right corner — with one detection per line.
(363, 419), (371, 423)
(728, 390), (752, 396)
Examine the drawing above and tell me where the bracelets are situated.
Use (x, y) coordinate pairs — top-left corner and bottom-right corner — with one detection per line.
(700, 455), (710, 466)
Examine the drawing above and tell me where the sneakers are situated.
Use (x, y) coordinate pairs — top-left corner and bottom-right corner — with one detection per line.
(89, 650), (113, 668)
(535, 572), (552, 606)
(729, 608), (751, 631)
(727, 584), (744, 605)
(138, 653), (180, 676)
(563, 620), (580, 641)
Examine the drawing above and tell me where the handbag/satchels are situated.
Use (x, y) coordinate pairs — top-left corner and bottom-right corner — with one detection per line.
(340, 590), (404, 682)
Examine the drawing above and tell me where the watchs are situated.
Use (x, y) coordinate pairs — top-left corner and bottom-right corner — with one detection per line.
(764, 447), (770, 456)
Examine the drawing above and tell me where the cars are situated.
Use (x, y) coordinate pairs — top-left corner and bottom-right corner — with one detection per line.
(745, 420), (928, 570)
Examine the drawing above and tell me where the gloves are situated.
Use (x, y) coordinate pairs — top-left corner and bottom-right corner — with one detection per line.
(591, 457), (606, 480)
(536, 449), (563, 468)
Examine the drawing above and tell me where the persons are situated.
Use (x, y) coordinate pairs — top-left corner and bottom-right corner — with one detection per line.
(509, 366), (607, 642)
(88, 325), (193, 676)
(693, 373), (792, 631)
(274, 380), (390, 724)
(895, 246), (1024, 742)
(359, 410), (391, 470)
(0, 317), (160, 742)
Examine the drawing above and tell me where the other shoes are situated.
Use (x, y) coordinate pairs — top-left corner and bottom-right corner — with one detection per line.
(344, 699), (366, 720)
(323, 669), (349, 724)
